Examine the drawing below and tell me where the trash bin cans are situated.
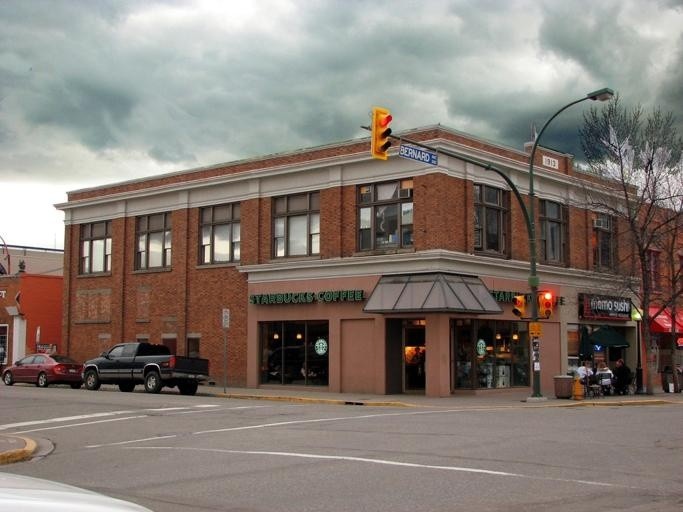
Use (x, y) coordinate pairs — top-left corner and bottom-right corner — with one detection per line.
(553, 375), (574, 399)
(660, 366), (683, 392)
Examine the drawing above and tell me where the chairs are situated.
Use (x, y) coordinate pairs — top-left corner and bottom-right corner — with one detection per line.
(581, 374), (637, 398)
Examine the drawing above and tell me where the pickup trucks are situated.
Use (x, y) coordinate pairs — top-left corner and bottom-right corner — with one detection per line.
(81, 341), (210, 394)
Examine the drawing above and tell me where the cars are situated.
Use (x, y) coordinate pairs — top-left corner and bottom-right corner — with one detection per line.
(1, 354), (83, 389)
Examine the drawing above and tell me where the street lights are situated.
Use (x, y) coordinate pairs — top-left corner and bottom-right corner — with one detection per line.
(631, 306), (645, 392)
(531, 86), (614, 397)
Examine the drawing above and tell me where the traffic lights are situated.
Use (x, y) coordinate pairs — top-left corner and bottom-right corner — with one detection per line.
(539, 291), (553, 318)
(512, 295), (525, 318)
(371, 108), (392, 161)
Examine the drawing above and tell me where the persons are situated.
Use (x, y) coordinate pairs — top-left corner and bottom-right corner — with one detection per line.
(576, 360), (595, 397)
(610, 358), (631, 395)
(45, 343), (55, 353)
(595, 362), (613, 395)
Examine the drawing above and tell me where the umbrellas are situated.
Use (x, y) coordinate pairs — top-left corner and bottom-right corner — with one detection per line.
(581, 325), (630, 367)
